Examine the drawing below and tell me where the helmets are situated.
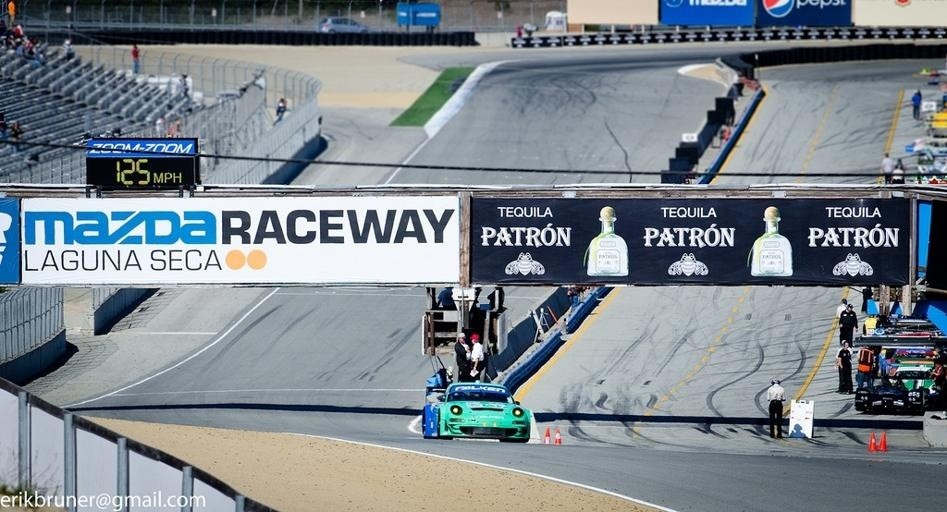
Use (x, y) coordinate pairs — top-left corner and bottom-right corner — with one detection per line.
(771, 378), (781, 384)
(840, 298), (847, 304)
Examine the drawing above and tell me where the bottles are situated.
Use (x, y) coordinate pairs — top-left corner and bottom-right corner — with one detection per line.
(586, 206), (629, 277)
(750, 207), (796, 278)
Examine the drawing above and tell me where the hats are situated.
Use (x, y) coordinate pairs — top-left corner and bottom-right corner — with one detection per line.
(457, 332), (466, 340)
(470, 333), (480, 342)
(848, 303), (854, 309)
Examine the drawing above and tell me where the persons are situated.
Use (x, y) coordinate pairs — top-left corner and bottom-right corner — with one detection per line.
(911, 87), (922, 121)
(130, 42), (141, 74)
(0, 112), (40, 165)
(156, 115), (183, 137)
(566, 285), (593, 312)
(487, 287), (505, 319)
(767, 379), (787, 440)
(882, 152), (907, 184)
(836, 298), (859, 395)
(179, 74), (189, 97)
(271, 98), (288, 127)
(454, 332), (486, 383)
(436, 287), (457, 310)
(931, 361), (946, 395)
(861, 286), (875, 314)
(1, 19), (75, 63)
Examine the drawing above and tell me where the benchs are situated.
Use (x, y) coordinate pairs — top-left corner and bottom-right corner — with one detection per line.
(0, 34), (206, 178)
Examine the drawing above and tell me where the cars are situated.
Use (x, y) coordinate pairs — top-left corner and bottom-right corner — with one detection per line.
(421, 382), (533, 443)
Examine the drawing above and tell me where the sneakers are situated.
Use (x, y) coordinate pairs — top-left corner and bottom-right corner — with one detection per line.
(836, 390), (851, 395)
(769, 433), (784, 438)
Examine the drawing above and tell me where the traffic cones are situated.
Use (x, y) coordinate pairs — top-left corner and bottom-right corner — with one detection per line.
(870, 433), (878, 452)
(554, 429), (561, 446)
(544, 429), (551, 444)
(878, 431), (888, 451)
